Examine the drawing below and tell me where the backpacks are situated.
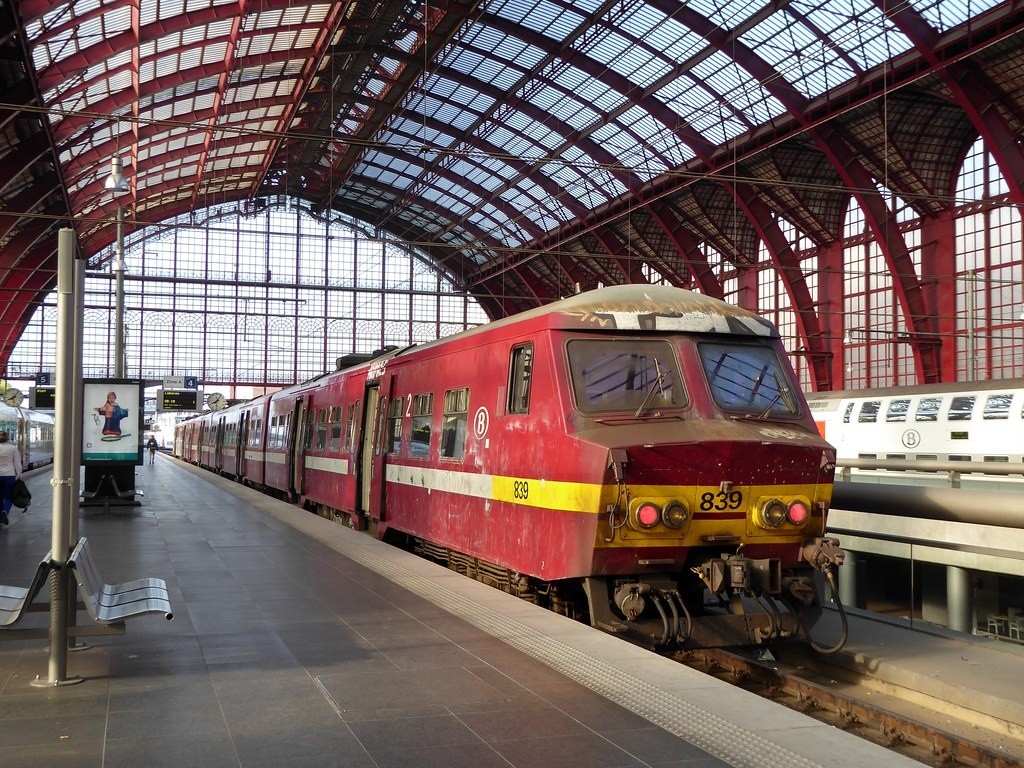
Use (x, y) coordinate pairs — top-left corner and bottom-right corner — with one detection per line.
(149, 440), (156, 451)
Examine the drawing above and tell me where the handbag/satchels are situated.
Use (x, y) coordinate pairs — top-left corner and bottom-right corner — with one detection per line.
(12, 478), (31, 508)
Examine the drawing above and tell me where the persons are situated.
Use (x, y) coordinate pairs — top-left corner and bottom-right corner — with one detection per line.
(93, 392), (128, 435)
(0, 431), (23, 526)
(147, 435), (158, 464)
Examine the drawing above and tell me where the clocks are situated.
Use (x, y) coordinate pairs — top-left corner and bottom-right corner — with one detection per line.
(207, 392), (226, 410)
(3, 388), (24, 407)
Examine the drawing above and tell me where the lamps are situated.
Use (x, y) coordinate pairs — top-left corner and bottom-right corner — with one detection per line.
(847, 361), (854, 372)
(843, 330), (853, 343)
(104, 121), (130, 193)
(112, 249), (129, 271)
(1019, 303), (1024, 319)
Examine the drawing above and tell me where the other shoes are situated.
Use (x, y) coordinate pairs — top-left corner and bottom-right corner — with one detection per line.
(149, 462), (151, 464)
(2, 510), (8, 525)
(152, 462), (154, 463)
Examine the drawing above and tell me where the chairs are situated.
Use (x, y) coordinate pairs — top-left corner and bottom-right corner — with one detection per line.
(987, 612), (1004, 635)
(0, 548), (52, 627)
(68, 537), (175, 624)
(78, 474), (144, 497)
(1010, 619), (1024, 639)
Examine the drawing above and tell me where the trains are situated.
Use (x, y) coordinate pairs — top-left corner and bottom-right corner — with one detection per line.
(173, 284), (847, 654)
(0, 401), (55, 471)
(803, 378), (1024, 491)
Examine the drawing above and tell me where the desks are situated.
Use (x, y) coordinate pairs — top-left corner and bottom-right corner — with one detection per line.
(992, 615), (1014, 620)
